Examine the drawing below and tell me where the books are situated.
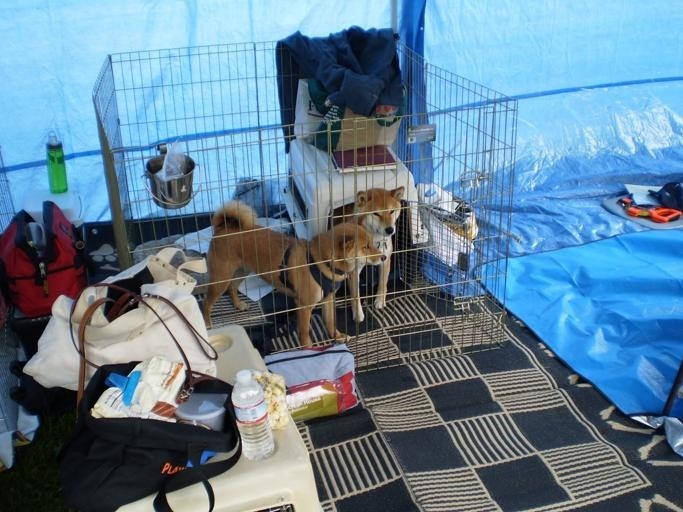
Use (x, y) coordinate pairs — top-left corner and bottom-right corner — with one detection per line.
(330, 145), (396, 174)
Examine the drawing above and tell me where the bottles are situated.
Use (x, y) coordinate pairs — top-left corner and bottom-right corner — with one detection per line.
(44, 131), (70, 195)
(229, 370), (279, 461)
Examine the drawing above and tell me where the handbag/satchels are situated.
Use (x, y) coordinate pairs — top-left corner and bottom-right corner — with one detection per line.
(58, 360), (243, 510)
(0, 200), (85, 318)
(22, 247), (216, 393)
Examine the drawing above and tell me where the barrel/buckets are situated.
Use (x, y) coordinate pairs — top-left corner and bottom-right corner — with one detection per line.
(140, 152), (200, 212)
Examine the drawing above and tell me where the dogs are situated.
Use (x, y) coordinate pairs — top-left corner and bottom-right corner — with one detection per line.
(328, 185), (405, 323)
(203, 198), (387, 350)
